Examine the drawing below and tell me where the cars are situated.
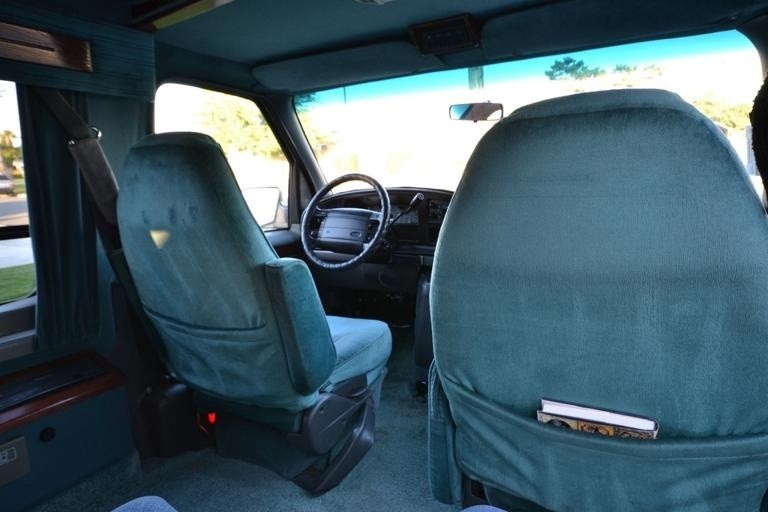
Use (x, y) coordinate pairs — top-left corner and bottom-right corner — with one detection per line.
(0, 172), (15, 194)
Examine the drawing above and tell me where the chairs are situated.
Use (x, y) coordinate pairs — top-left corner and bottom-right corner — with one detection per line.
(422, 87), (767, 511)
(117, 128), (396, 499)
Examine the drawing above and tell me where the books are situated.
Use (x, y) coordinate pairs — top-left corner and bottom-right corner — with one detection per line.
(535, 396), (660, 440)
